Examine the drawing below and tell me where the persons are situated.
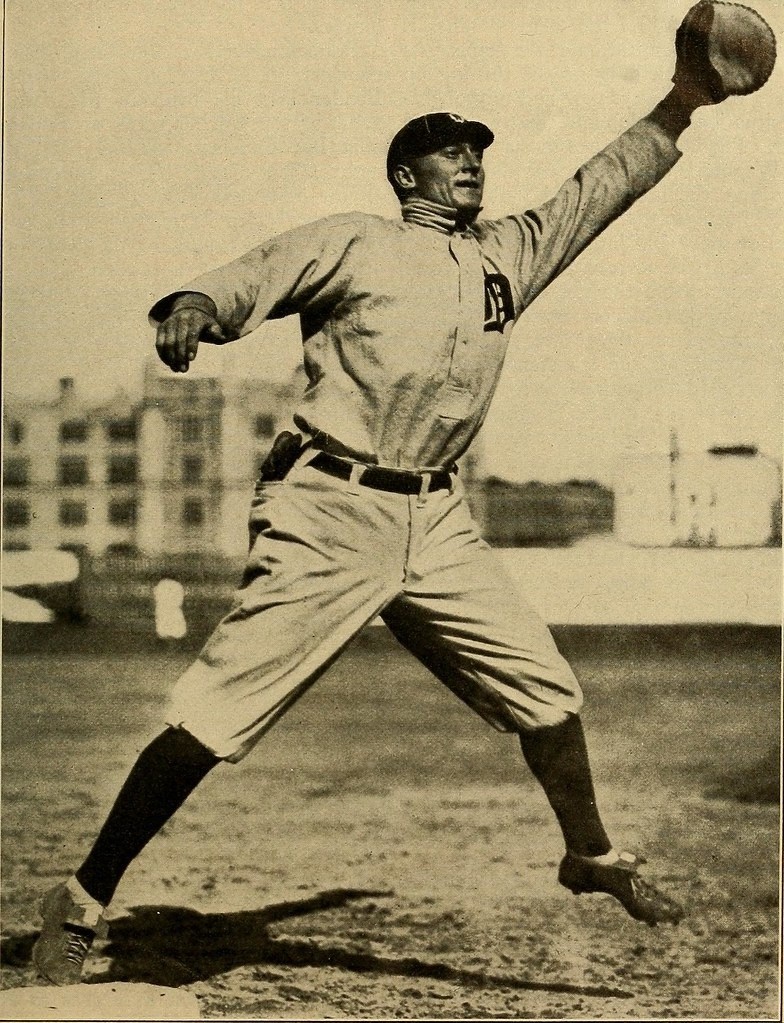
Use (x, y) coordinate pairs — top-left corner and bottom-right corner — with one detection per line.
(26, 56), (727, 952)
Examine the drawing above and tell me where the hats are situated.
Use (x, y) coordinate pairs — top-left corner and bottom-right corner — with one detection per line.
(387, 113), (494, 179)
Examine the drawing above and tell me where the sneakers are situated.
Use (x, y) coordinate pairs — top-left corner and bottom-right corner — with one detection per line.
(31, 880), (109, 988)
(557, 850), (685, 927)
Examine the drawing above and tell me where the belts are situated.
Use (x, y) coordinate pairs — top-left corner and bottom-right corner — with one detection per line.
(301, 441), (454, 494)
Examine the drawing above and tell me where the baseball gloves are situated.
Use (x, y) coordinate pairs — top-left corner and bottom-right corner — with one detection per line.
(670, 0), (778, 104)
